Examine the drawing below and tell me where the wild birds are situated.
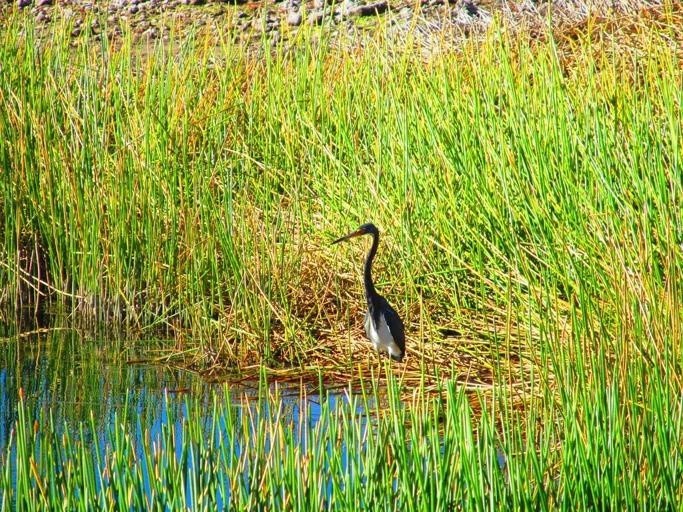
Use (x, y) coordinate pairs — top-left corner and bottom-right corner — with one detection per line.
(328, 222), (406, 400)
